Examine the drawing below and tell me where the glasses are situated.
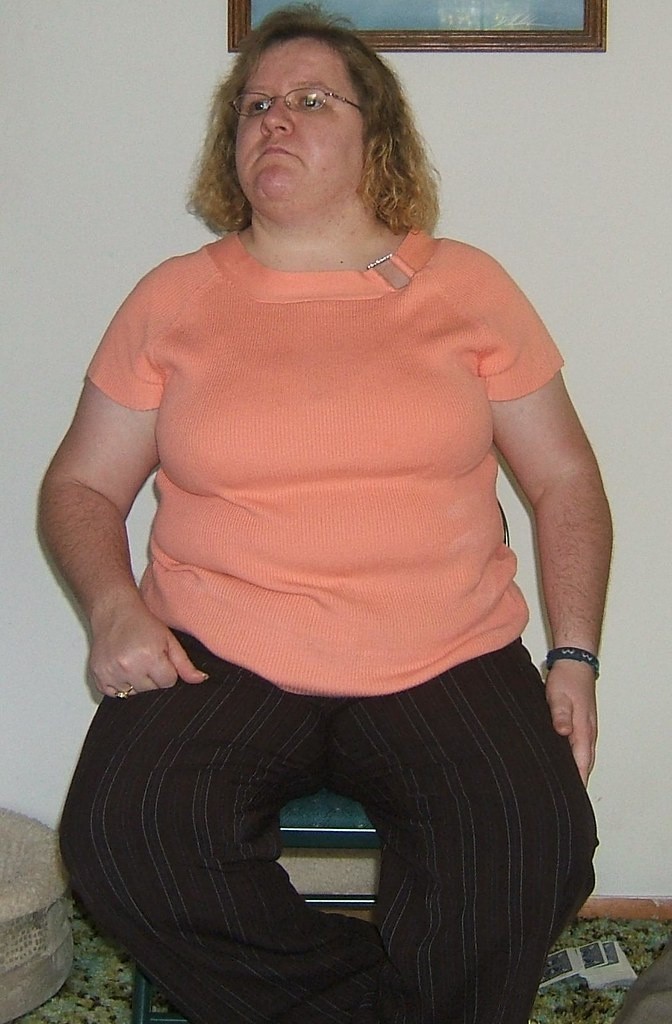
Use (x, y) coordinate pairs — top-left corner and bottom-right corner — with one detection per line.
(229, 87), (362, 117)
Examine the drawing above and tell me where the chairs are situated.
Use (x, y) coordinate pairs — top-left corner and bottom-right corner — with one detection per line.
(130, 791), (379, 1024)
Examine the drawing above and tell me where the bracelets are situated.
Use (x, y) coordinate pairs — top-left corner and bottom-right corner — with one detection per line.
(547, 647), (600, 679)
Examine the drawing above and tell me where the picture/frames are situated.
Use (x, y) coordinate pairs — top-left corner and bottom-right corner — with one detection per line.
(228, 1), (610, 51)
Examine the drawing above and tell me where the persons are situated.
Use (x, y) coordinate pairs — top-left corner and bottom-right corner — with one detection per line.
(38, 1), (615, 1024)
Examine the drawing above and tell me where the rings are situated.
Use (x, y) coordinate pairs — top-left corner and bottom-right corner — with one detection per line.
(115, 685), (133, 699)
